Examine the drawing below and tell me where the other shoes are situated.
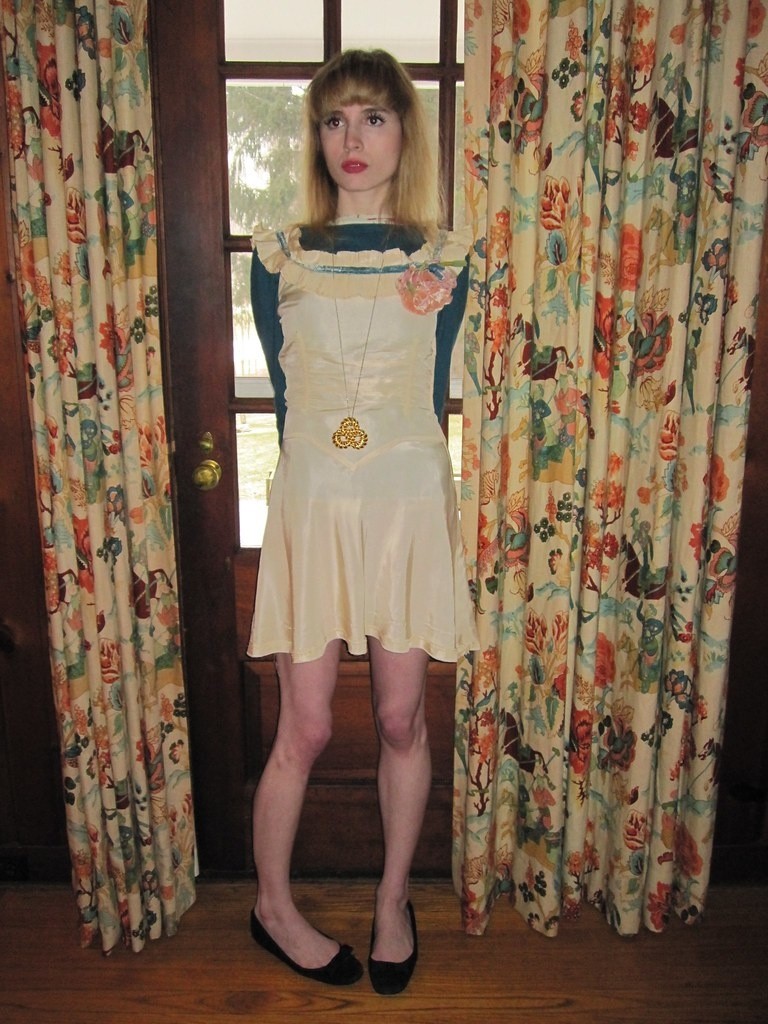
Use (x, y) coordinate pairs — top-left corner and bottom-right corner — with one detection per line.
(368, 899), (418, 995)
(249, 905), (364, 986)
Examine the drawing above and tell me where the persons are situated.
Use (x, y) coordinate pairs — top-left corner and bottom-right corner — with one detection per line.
(246, 49), (481, 993)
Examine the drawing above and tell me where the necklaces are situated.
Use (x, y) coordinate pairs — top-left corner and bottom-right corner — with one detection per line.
(332, 226), (393, 451)
